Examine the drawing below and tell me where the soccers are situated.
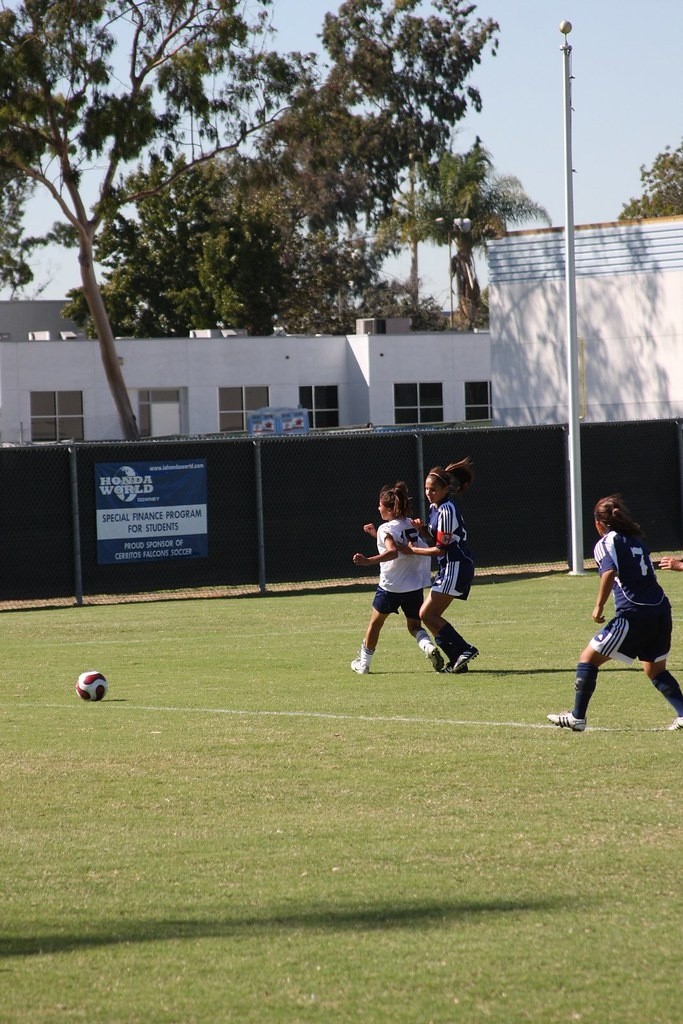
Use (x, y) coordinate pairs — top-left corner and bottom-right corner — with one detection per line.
(75, 670), (108, 702)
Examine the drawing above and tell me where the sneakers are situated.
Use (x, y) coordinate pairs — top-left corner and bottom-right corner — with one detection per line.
(425, 643), (444, 671)
(437, 660), (469, 673)
(351, 660), (369, 674)
(668, 716), (683, 731)
(546, 710), (587, 731)
(454, 646), (480, 671)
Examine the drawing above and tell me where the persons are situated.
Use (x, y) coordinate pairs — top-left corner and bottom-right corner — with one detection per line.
(351, 482), (444, 674)
(396, 458), (479, 673)
(547, 498), (683, 732)
(659, 556), (683, 571)
